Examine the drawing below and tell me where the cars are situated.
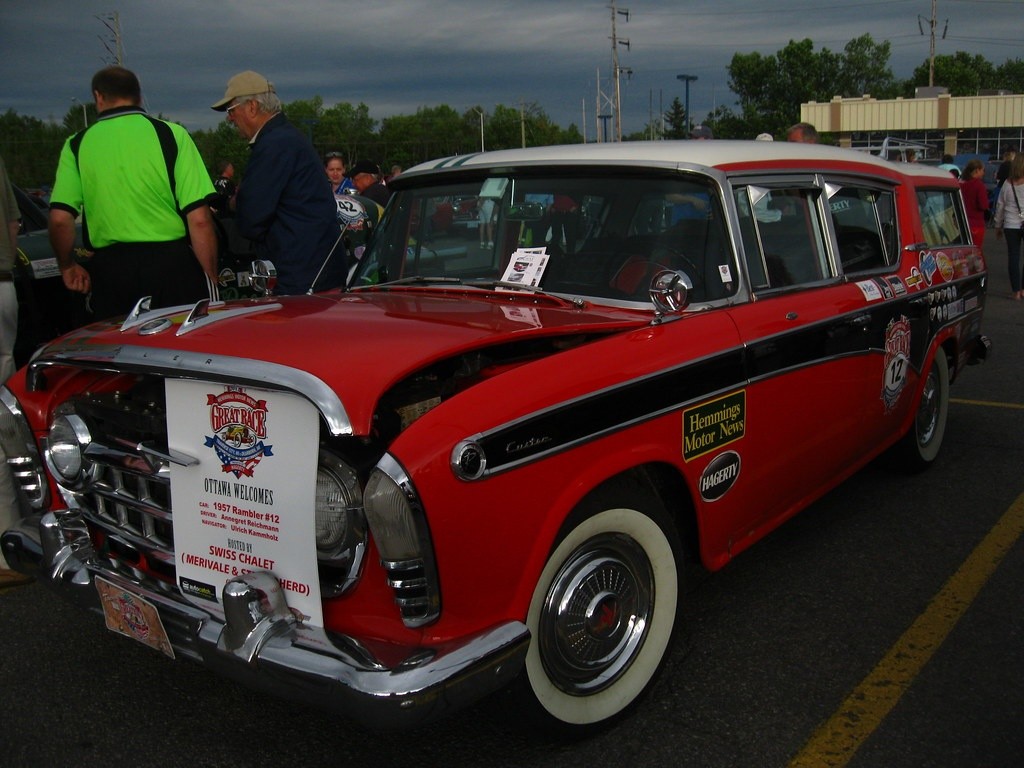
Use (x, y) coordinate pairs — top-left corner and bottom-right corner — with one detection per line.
(450, 194), (578, 240)
(409, 195), (480, 238)
(11, 182), (474, 369)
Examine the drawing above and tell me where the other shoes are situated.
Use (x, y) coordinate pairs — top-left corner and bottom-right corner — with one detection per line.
(480, 243), (492, 250)
(1011, 289), (1024, 300)
(0, 567), (37, 588)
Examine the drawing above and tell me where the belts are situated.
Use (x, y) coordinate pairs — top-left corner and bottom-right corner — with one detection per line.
(557, 210), (568, 215)
(0, 271), (14, 281)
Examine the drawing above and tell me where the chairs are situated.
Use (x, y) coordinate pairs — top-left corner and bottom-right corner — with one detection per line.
(538, 218), (883, 303)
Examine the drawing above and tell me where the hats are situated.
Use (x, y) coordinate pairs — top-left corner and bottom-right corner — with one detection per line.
(342, 159), (380, 177)
(688, 126), (713, 138)
(211, 71), (275, 112)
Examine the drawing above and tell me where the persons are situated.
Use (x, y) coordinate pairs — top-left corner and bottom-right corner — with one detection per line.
(474, 187), (578, 249)
(959, 159), (989, 249)
(49, 66), (222, 324)
(939, 148), (1016, 228)
(211, 70), (346, 296)
(994, 152), (1024, 300)
(896, 149), (919, 164)
(324, 151), (411, 211)
(1, 157), (38, 587)
(666, 121), (826, 229)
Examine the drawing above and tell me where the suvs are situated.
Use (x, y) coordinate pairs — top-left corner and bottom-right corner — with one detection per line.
(1, 140), (992, 741)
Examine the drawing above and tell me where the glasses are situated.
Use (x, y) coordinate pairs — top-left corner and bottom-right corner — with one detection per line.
(226, 103), (240, 112)
(326, 151), (343, 157)
